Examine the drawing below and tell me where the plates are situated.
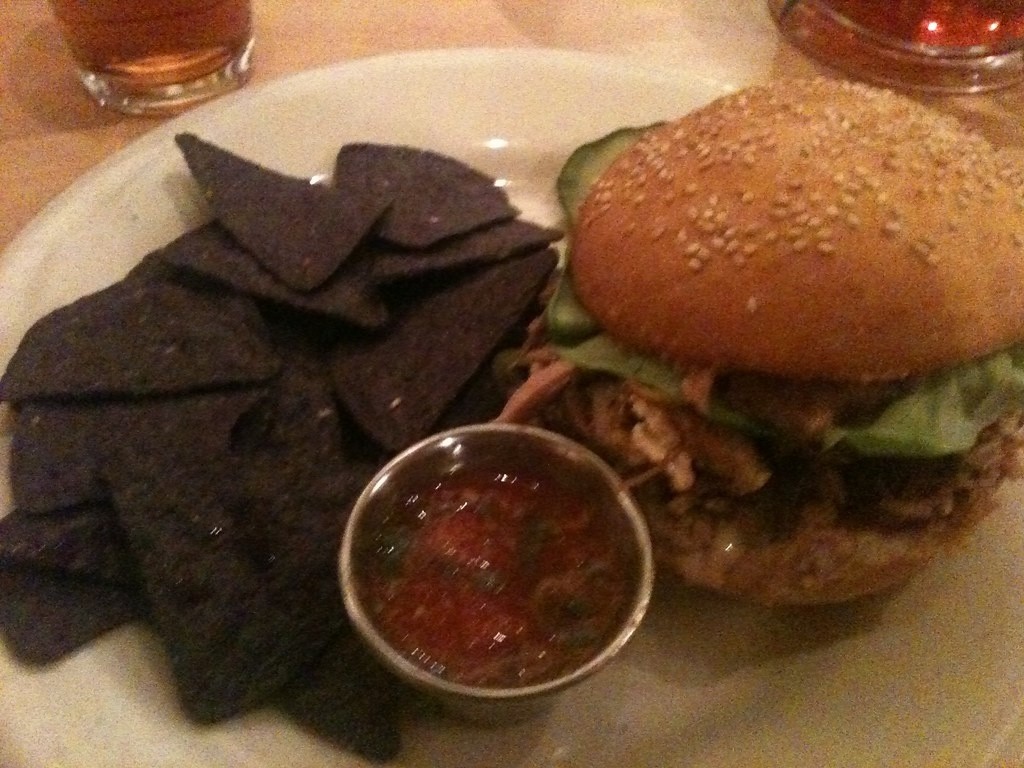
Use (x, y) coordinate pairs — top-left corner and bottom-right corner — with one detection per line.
(0, 50), (1024, 768)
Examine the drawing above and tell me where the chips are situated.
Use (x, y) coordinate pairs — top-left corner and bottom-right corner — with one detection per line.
(0, 132), (564, 765)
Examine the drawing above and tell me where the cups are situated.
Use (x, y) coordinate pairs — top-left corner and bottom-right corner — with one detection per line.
(336, 424), (652, 725)
(760, 0), (1024, 97)
(48, 0), (256, 116)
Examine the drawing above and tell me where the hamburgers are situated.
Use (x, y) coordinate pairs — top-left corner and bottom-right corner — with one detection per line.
(487, 77), (1023, 606)
